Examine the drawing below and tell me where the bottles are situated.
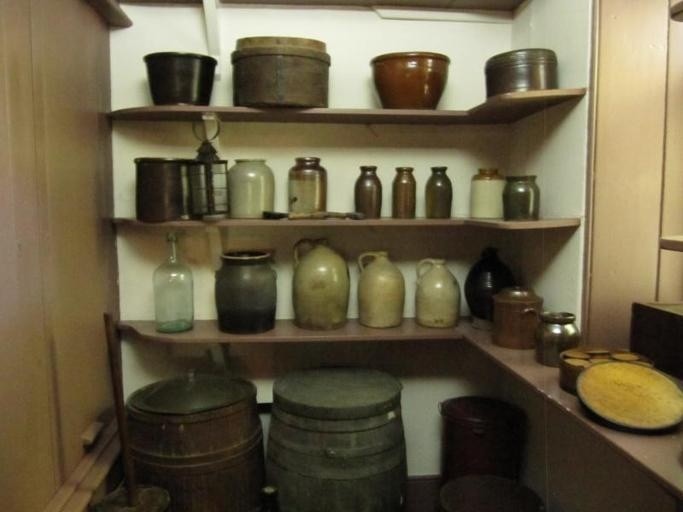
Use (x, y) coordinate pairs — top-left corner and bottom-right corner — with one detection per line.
(152, 231), (194, 332)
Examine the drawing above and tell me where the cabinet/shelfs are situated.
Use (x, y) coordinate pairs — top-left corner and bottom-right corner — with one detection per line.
(468, 337), (682, 511)
(651, 0), (683, 256)
(104, 85), (588, 347)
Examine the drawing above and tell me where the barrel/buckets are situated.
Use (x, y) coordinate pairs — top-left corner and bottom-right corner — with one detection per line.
(267, 363), (407, 512)
(121, 371), (263, 511)
